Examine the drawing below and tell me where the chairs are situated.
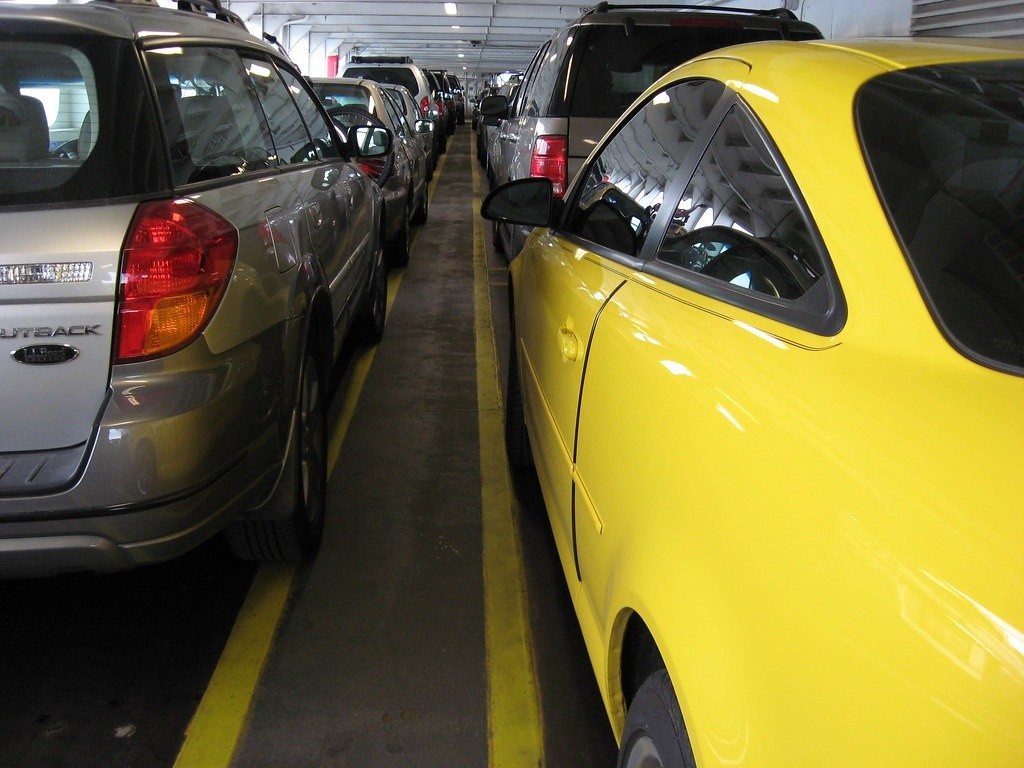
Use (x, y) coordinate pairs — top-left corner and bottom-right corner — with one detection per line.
(342, 104), (369, 124)
(0, 94), (50, 163)
(179, 95), (246, 163)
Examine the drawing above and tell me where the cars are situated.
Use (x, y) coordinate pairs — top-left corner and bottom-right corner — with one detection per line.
(261, 0), (829, 271)
(476, 37), (1024, 768)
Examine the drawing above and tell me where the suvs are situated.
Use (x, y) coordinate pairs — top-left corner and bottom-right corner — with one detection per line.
(0, 0), (397, 581)
